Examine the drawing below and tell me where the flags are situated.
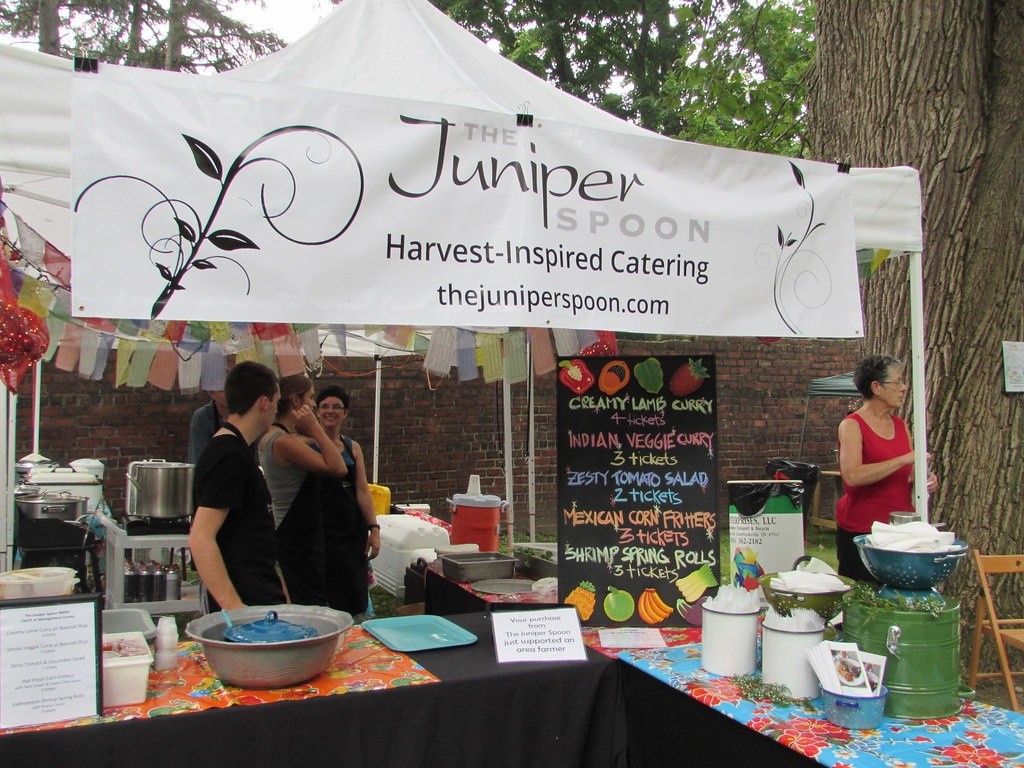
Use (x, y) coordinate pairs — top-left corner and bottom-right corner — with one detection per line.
(364, 325), (598, 382)
(10, 217), (347, 391)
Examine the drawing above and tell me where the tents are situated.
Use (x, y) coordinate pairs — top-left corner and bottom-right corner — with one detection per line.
(0, 0), (928, 570)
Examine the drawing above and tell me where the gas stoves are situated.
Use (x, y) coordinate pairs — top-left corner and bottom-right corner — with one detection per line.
(121, 515), (191, 536)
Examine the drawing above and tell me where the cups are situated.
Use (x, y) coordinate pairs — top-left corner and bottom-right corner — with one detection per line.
(702, 604), (760, 675)
(761, 618), (824, 701)
(153, 615), (180, 671)
(467, 475), (480, 494)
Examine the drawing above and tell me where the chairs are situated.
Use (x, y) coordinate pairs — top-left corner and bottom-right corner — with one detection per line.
(968, 549), (1024, 713)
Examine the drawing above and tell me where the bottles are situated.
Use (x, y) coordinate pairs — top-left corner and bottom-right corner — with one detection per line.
(125, 559), (182, 603)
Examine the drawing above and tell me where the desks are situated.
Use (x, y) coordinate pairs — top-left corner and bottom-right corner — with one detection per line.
(0, 547), (1024, 768)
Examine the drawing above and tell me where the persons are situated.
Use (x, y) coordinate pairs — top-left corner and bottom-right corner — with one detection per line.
(257, 374), (381, 613)
(836, 355), (938, 583)
(188, 361), (290, 614)
(188, 368), (231, 464)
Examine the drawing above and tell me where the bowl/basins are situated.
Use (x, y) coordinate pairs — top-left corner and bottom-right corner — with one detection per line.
(185, 603), (355, 687)
(819, 683), (887, 729)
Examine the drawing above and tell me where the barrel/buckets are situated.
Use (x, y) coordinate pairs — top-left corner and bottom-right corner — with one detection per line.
(843, 587), (963, 719)
(447, 494), (509, 553)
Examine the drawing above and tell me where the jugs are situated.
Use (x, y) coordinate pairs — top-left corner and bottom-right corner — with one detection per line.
(0, 567), (80, 599)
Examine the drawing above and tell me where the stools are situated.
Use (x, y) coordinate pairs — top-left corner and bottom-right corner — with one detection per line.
(19, 543), (104, 595)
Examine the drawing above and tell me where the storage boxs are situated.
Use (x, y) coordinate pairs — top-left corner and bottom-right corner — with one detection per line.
(102, 631), (154, 708)
(102, 608), (157, 641)
(367, 530), (438, 598)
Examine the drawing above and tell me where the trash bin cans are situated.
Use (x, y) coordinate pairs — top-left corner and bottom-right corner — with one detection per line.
(766, 460), (820, 546)
(727, 480), (812, 592)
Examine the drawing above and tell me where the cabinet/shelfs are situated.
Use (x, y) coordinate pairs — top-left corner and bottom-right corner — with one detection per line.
(105, 517), (207, 621)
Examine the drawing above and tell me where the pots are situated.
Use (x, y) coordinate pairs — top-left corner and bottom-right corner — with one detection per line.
(762, 557), (852, 624)
(16, 490), (88, 526)
(127, 458), (196, 519)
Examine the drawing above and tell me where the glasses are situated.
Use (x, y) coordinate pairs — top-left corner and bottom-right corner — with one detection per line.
(881, 378), (903, 387)
(319, 405), (344, 409)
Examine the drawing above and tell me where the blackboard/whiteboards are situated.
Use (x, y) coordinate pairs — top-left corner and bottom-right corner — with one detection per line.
(555, 355), (722, 627)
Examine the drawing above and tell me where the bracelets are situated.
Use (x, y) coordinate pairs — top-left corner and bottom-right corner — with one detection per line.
(369, 523), (380, 530)
